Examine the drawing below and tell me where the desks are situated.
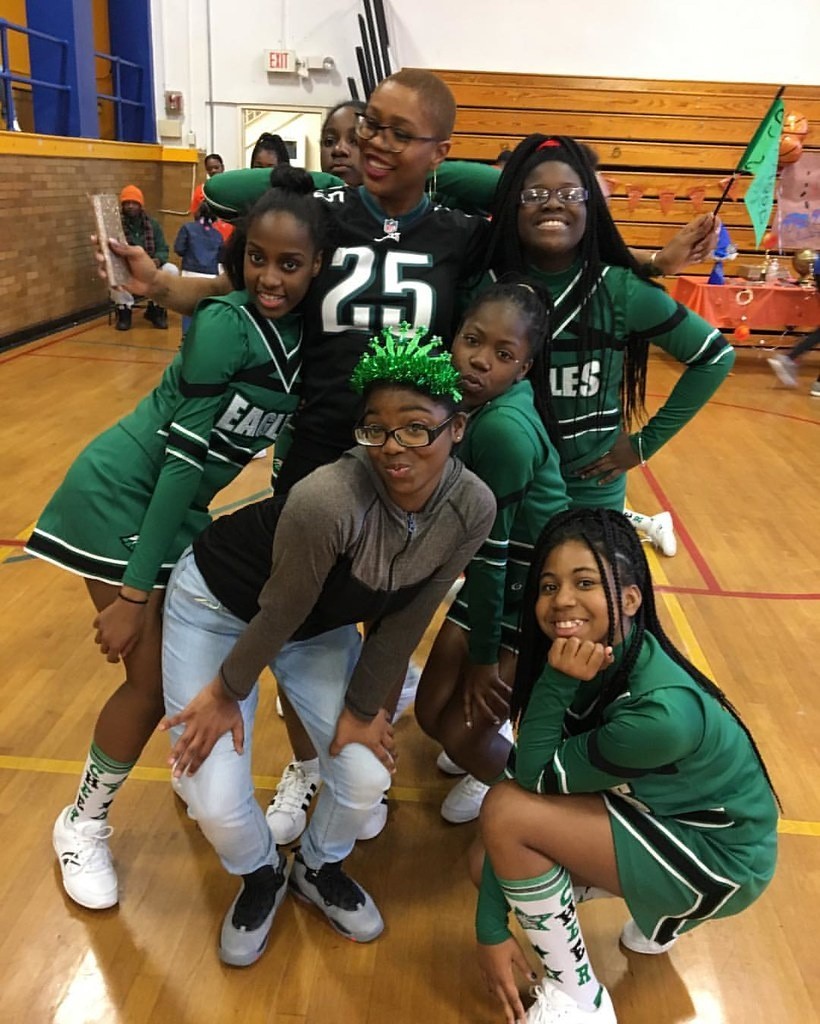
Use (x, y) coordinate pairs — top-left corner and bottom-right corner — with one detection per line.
(672, 275), (820, 328)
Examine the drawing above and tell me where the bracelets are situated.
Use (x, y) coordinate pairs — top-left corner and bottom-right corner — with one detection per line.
(651, 250), (664, 277)
(118, 591), (147, 605)
(639, 437), (643, 464)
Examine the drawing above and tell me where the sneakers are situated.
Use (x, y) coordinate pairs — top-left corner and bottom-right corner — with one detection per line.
(441, 774), (490, 823)
(514, 978), (617, 1024)
(620, 918), (675, 954)
(288, 845), (383, 942)
(219, 852), (290, 966)
(265, 762), (321, 844)
(437, 718), (515, 774)
(52, 804), (118, 909)
(356, 791), (388, 840)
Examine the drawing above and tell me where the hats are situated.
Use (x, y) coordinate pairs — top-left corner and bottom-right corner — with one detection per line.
(120, 185), (143, 207)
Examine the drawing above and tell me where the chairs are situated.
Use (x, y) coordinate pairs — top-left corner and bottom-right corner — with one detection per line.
(108, 288), (148, 327)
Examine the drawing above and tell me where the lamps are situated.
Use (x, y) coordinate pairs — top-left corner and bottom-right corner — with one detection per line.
(297, 55), (336, 78)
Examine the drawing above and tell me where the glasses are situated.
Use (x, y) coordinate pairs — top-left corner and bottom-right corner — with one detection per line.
(353, 414), (454, 446)
(354, 112), (441, 153)
(521, 187), (588, 203)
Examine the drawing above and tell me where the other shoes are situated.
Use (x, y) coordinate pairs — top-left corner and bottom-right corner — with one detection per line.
(810, 381), (820, 396)
(143, 301), (168, 329)
(116, 309), (131, 329)
(641, 511), (676, 556)
(766, 355), (796, 385)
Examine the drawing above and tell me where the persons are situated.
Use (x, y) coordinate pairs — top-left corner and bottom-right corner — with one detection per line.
(484, 150), (512, 220)
(475, 506), (784, 1024)
(202, 101), (518, 724)
(413, 279), (572, 824)
(22, 164), (337, 910)
(113, 132), (289, 349)
(767, 256), (820, 396)
(90, 69), (723, 845)
(160, 321), (498, 967)
(624, 511), (677, 556)
(437, 133), (737, 617)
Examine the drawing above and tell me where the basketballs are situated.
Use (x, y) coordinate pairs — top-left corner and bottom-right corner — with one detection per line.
(773, 112), (808, 167)
